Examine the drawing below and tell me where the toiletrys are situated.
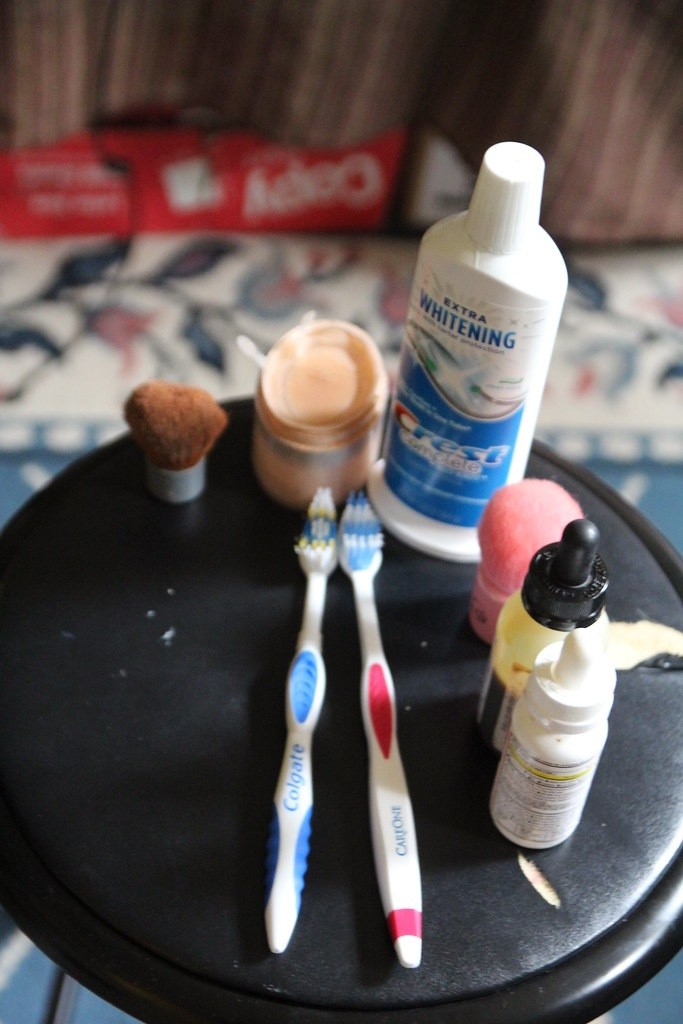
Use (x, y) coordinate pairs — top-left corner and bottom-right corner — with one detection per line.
(377, 142), (570, 535)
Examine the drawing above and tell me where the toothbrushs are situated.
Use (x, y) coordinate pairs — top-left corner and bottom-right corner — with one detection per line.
(335, 486), (427, 972)
(259, 484), (347, 957)
